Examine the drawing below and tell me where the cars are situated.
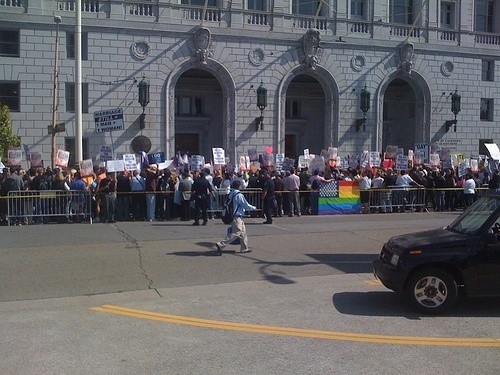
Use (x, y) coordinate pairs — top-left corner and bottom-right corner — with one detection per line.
(370, 196), (500, 316)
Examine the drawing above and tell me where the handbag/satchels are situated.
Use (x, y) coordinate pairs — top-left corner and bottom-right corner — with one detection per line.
(273, 199), (279, 210)
(201, 193), (210, 208)
(227, 227), (240, 245)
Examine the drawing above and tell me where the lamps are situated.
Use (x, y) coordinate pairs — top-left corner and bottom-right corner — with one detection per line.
(445, 84), (462, 133)
(137, 75), (151, 130)
(255, 78), (268, 133)
(355, 82), (370, 133)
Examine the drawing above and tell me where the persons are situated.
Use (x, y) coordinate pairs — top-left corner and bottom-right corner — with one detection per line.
(488, 168), (500, 193)
(191, 173), (216, 226)
(0, 155), (500, 226)
(99, 172), (117, 224)
(215, 180), (257, 257)
(462, 176), (475, 209)
(260, 173), (275, 224)
(284, 169), (302, 217)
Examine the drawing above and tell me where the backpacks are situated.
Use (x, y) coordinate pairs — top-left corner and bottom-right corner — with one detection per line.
(160, 177), (171, 198)
(220, 191), (241, 225)
(311, 179), (318, 190)
(37, 176), (48, 190)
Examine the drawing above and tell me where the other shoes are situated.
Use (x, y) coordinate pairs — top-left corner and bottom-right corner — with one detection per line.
(240, 250), (252, 253)
(298, 212), (301, 217)
(192, 221), (200, 225)
(288, 213), (295, 217)
(203, 220), (207, 226)
(263, 220), (272, 224)
(216, 242), (222, 256)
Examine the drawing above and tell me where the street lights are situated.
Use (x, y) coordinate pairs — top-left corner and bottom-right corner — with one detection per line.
(51, 15), (62, 171)
